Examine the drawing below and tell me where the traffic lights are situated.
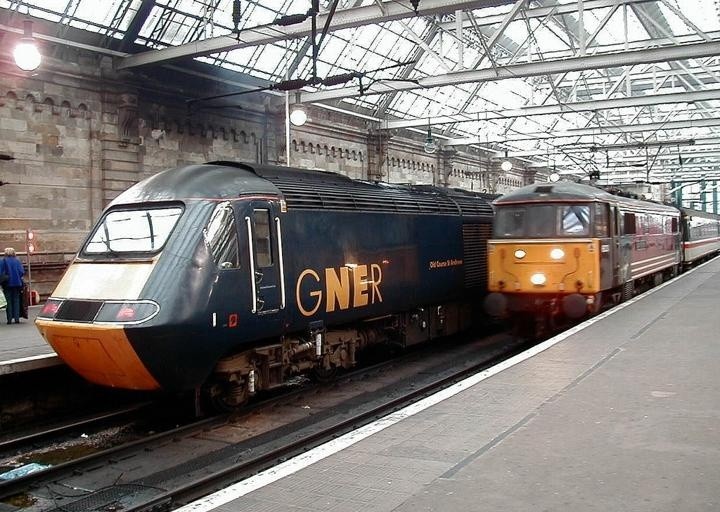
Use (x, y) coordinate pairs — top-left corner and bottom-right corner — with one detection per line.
(26, 229), (34, 257)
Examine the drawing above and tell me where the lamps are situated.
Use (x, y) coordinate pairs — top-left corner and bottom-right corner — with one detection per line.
(290, 91), (307, 126)
(550, 166), (560, 182)
(13, 8), (42, 70)
(424, 118), (435, 153)
(501, 151), (512, 171)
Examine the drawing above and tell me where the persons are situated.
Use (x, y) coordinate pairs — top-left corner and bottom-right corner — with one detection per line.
(0, 248), (26, 323)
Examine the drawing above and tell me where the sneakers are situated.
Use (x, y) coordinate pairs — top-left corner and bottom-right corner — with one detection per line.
(7, 319), (19, 324)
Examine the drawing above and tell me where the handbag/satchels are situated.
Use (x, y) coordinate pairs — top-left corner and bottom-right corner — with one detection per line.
(20, 296), (28, 320)
(0, 275), (10, 285)
